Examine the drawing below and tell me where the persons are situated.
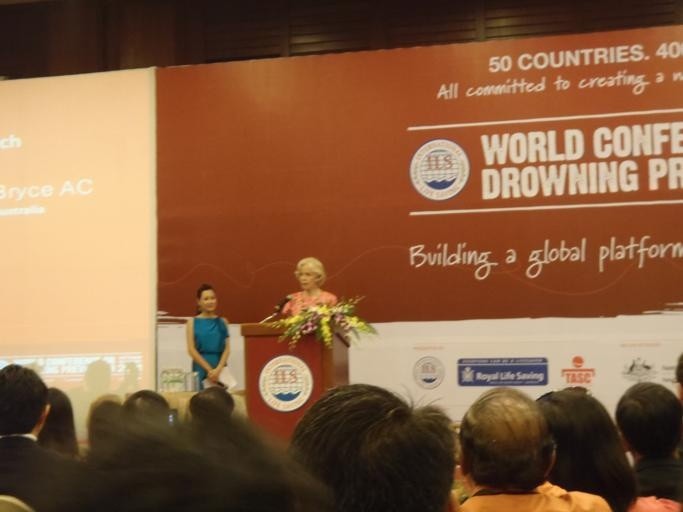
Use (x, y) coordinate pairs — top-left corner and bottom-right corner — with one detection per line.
(184, 284), (231, 391)
(1, 358), (683, 512)
(280, 256), (340, 320)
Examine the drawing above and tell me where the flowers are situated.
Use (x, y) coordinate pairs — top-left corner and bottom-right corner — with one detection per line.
(270, 293), (378, 352)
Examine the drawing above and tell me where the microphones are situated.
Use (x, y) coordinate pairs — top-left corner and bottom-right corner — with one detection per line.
(274, 296), (292, 311)
